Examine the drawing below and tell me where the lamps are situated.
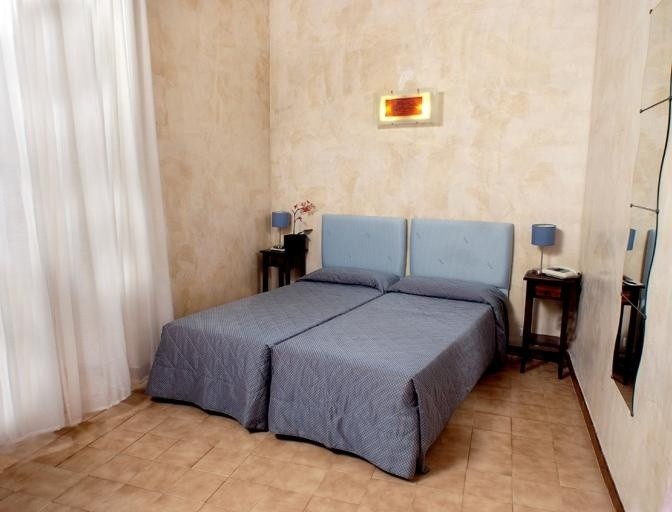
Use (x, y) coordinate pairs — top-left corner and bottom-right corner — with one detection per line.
(374, 88), (441, 129)
(531, 223), (556, 273)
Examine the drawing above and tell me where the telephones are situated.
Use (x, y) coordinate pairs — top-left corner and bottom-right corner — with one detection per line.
(542, 266), (580, 279)
(623, 275), (642, 286)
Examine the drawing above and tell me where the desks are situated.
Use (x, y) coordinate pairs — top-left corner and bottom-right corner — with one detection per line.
(519, 271), (582, 380)
(613, 283), (644, 384)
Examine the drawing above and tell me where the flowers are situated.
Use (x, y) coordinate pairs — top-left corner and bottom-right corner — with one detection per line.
(290, 199), (317, 234)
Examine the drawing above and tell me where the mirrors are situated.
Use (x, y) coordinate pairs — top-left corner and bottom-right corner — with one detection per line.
(609, 0), (671, 417)
